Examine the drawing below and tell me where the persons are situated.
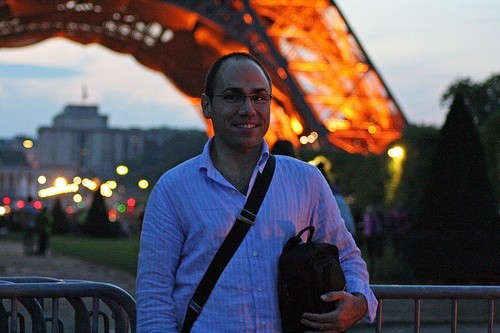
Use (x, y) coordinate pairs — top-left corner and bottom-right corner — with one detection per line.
(0, 183), (119, 257)
(363, 202), (384, 257)
(135, 51), (381, 333)
(390, 200), (409, 254)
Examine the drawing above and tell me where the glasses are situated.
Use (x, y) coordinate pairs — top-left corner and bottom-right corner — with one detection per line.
(210, 93), (273, 105)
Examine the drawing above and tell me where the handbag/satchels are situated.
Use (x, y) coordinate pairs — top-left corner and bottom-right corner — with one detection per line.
(277, 224), (347, 333)
